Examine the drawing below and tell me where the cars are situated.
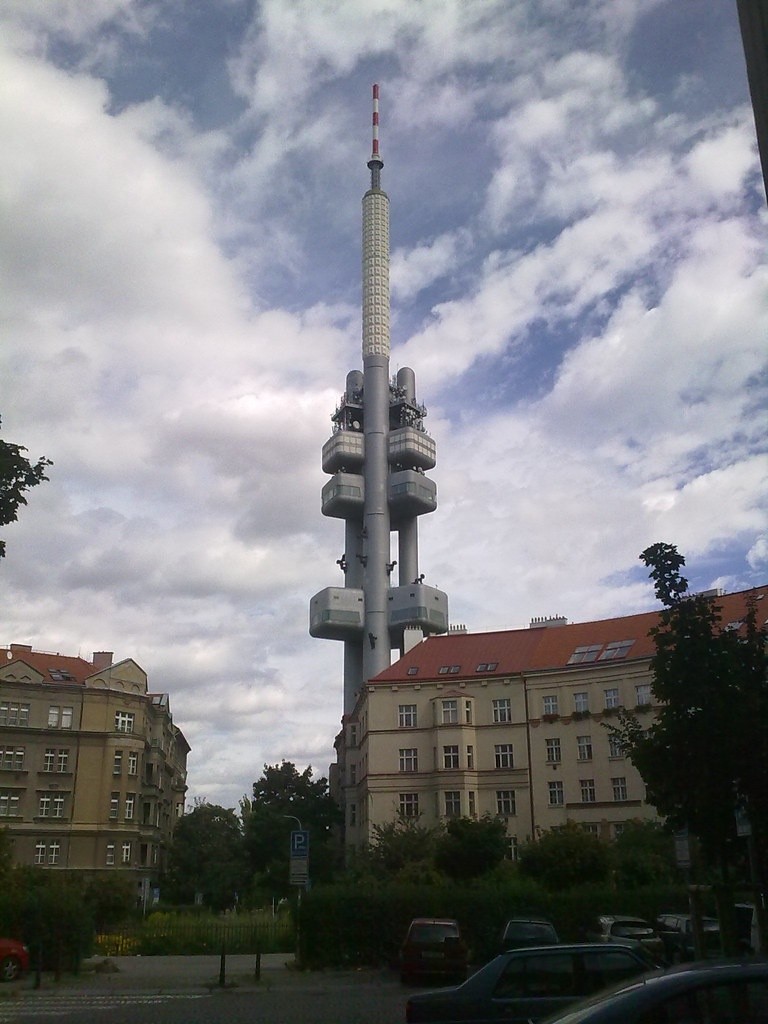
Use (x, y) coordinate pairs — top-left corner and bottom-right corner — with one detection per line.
(406, 935), (715, 1024)
(495, 917), (560, 950)
(652, 912), (719, 950)
(582, 915), (664, 966)
(0, 937), (29, 981)
(534, 955), (768, 1024)
(398, 919), (467, 983)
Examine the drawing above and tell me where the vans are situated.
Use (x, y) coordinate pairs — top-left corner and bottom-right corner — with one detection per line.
(733, 903), (766, 954)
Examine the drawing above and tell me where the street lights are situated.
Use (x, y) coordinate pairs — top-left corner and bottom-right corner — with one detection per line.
(283, 814), (301, 928)
